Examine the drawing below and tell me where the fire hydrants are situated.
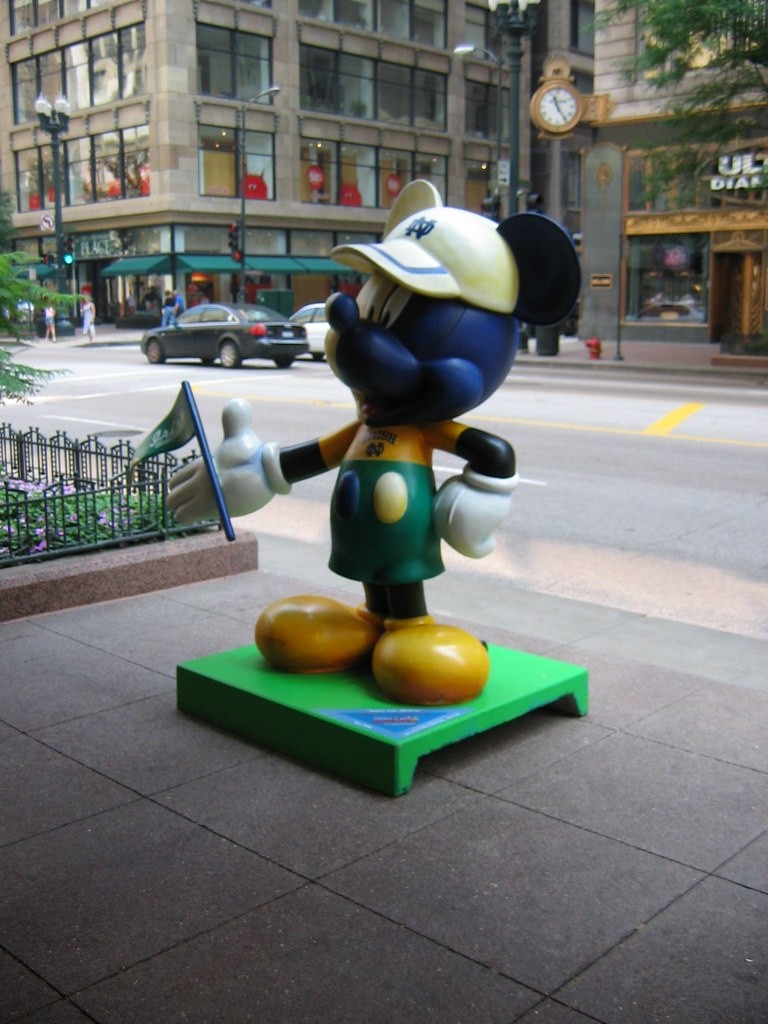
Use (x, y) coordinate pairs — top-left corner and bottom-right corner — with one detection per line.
(587, 338), (603, 360)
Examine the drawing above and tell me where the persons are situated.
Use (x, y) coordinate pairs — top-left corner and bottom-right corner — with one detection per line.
(160, 290), (185, 327)
(44, 306), (57, 344)
(79, 299), (96, 343)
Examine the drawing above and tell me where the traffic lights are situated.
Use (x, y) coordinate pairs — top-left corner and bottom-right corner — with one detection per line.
(227, 223), (243, 263)
(480, 194), (500, 224)
(525, 191), (545, 216)
(60, 233), (74, 280)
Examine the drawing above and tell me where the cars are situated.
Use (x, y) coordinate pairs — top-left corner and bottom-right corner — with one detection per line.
(4, 301), (37, 328)
(287, 303), (332, 359)
(140, 301), (310, 368)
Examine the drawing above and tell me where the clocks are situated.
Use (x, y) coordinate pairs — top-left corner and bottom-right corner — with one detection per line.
(535, 84), (580, 132)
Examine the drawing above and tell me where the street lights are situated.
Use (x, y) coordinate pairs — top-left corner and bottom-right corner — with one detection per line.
(239, 86), (281, 303)
(34, 90), (75, 337)
(487, 0), (544, 354)
(452, 43), (504, 192)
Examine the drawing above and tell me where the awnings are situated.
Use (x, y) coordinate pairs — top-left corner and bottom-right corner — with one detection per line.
(12, 263), (58, 281)
(100, 255), (171, 277)
(175, 253), (356, 273)
